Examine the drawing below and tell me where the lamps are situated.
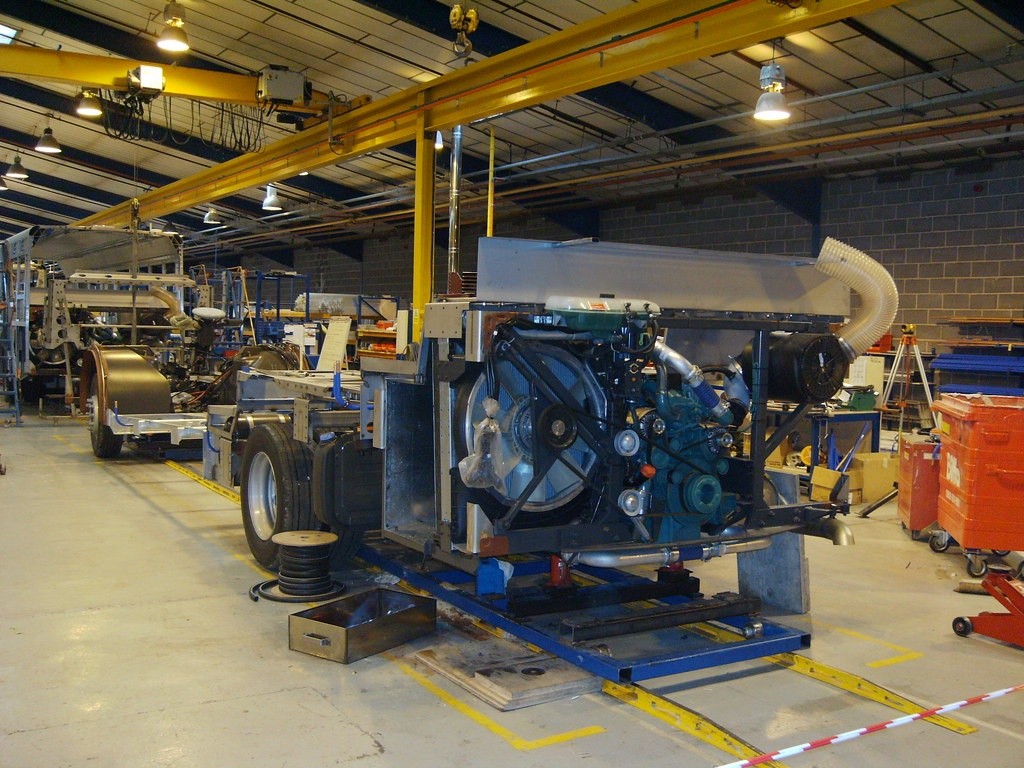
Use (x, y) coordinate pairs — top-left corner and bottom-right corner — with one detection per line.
(0, 174), (8, 190)
(436, 131), (443, 149)
(754, 36), (791, 120)
(6, 151), (29, 179)
(35, 112), (62, 153)
(157, 0), (189, 51)
(76, 86), (102, 116)
(162, 219), (178, 234)
(203, 207), (221, 224)
(262, 183), (282, 211)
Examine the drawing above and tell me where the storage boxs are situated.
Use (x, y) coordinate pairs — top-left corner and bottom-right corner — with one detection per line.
(810, 464), (863, 506)
(843, 452), (900, 503)
(288, 587), (436, 665)
(868, 334), (893, 352)
(742, 427), (793, 469)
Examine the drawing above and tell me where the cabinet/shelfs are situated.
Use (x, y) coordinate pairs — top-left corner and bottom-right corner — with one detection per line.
(355, 328), (397, 361)
(862, 348), (937, 432)
